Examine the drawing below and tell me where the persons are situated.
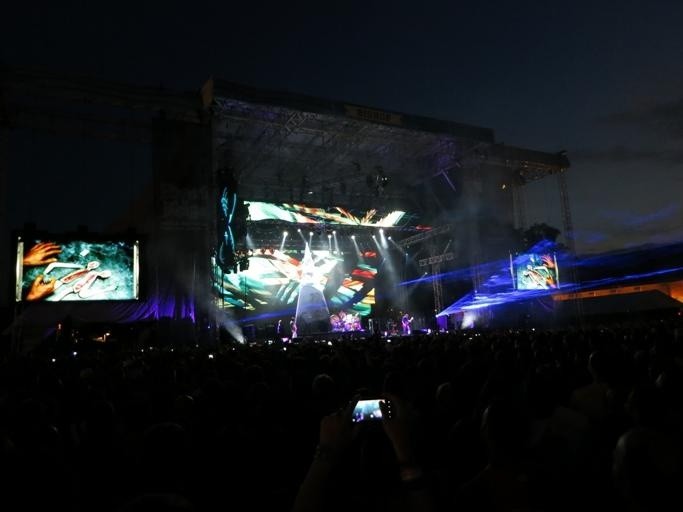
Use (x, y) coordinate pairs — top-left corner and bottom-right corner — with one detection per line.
(15, 240), (64, 305)
(286, 314), (302, 340)
(400, 311), (416, 339)
(534, 254), (557, 292)
(1, 332), (683, 511)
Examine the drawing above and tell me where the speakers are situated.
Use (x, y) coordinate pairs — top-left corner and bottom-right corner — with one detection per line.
(266, 324), (275, 339)
(437, 315), (449, 330)
(292, 338), (302, 344)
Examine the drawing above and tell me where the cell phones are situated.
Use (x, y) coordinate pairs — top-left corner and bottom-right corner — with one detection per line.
(350, 396), (393, 424)
